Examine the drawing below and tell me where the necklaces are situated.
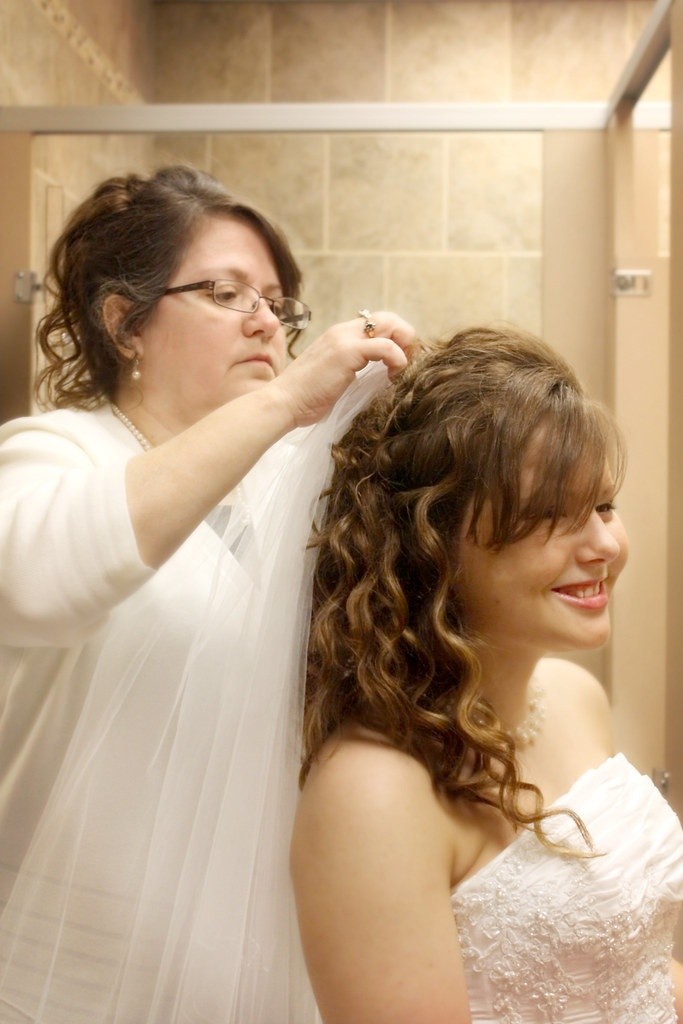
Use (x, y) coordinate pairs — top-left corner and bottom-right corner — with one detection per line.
(505, 685), (547, 744)
(106, 399), (155, 453)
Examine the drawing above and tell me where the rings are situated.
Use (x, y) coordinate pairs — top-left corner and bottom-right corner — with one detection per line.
(357, 308), (377, 337)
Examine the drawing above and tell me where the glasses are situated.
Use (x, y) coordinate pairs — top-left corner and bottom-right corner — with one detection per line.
(163, 278), (311, 330)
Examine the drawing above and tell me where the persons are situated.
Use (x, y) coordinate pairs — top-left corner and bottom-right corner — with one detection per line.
(1, 160), (416, 1024)
(290, 327), (683, 1024)
